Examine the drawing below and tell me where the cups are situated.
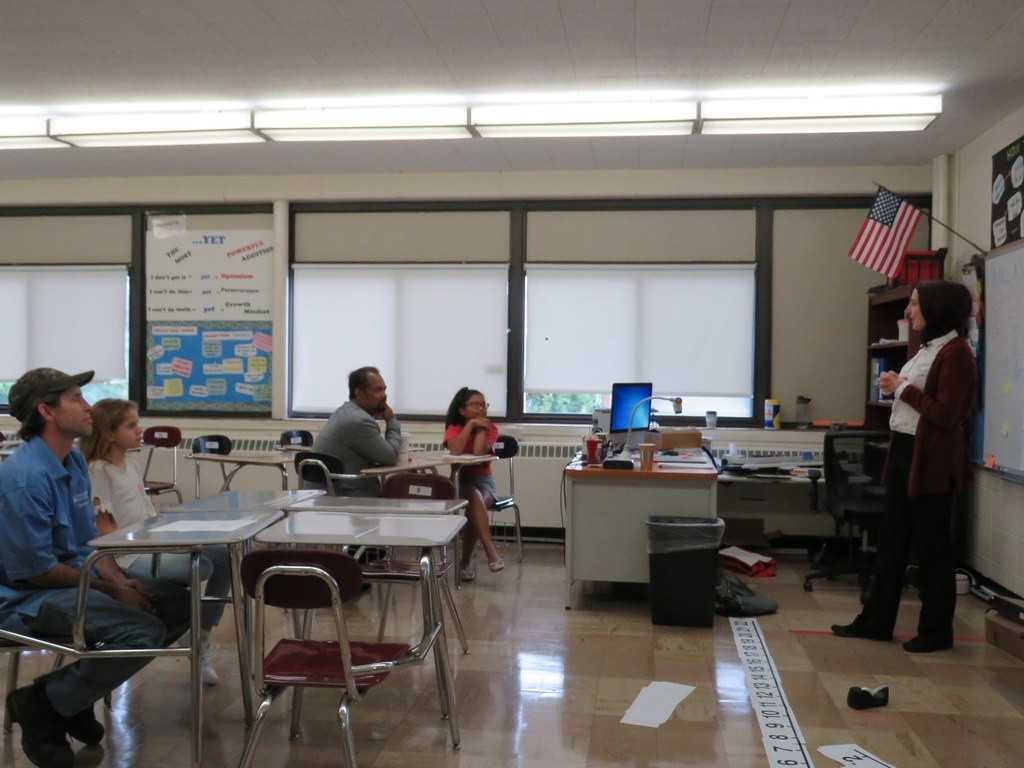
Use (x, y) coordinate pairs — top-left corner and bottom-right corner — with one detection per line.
(898, 320), (908, 342)
(705, 411), (717, 429)
(574, 441), (608, 464)
(638, 444), (656, 471)
(397, 432), (411, 465)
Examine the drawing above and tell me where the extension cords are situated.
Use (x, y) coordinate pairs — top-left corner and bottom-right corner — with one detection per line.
(971, 585), (991, 601)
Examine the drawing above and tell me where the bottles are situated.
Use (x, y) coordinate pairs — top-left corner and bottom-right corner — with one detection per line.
(796, 396), (813, 429)
(764, 399), (781, 430)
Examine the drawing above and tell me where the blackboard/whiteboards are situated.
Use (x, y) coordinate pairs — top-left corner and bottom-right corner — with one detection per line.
(982, 237), (1024, 480)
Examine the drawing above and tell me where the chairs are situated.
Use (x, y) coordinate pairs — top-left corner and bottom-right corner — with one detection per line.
(352, 472), (470, 652)
(295, 451), (382, 559)
(143, 426), (183, 505)
(280, 430), (313, 469)
(803, 429), (890, 592)
(191, 435), (232, 500)
(238, 550), (464, 768)
(460, 434), (523, 563)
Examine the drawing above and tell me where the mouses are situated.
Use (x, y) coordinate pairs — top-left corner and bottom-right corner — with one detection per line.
(660, 450), (678, 456)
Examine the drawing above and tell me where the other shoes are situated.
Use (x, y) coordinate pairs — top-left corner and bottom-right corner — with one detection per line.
(904, 628), (955, 652)
(831, 611), (894, 641)
(489, 558), (504, 572)
(461, 568), (475, 581)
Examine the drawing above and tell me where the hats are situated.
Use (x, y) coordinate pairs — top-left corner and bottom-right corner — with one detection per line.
(8, 367), (96, 423)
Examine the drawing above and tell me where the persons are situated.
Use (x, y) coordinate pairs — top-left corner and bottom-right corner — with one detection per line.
(831, 280), (980, 653)
(444, 387), (504, 580)
(79, 398), (239, 685)
(301, 366), (401, 560)
(0, 367), (192, 768)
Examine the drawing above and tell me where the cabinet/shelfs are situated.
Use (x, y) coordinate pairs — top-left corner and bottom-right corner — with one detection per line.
(862, 282), (928, 485)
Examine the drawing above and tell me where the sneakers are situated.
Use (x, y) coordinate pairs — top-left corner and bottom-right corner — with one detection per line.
(6, 683), (76, 768)
(68, 704), (104, 745)
(177, 629), (220, 684)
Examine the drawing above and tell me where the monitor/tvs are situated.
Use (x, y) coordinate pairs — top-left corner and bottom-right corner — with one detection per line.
(609, 383), (653, 434)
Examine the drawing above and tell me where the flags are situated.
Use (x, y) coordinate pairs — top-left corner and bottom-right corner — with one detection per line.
(847, 186), (924, 279)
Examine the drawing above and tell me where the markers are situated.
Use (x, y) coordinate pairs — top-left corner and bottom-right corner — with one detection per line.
(998, 466), (1008, 472)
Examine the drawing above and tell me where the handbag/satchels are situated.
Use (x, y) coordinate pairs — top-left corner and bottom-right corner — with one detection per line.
(716, 568), (780, 614)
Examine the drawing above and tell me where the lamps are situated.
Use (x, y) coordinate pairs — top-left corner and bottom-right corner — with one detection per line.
(617, 397), (683, 461)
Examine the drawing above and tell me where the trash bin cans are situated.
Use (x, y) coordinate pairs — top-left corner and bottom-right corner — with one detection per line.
(646, 515), (724, 628)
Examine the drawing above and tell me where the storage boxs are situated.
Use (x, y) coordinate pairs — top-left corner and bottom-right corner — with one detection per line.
(984, 607), (1024, 661)
(887, 249), (943, 285)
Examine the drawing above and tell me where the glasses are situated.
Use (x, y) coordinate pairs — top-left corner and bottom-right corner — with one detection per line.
(464, 401), (490, 408)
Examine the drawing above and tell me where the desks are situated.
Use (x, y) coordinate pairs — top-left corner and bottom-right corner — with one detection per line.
(159, 490), (328, 638)
(716, 464), (860, 555)
(360, 454), (455, 562)
(287, 497), (470, 516)
(74, 508), (283, 768)
(414, 450), (501, 592)
(563, 451), (719, 608)
(183, 452), (300, 515)
(253, 510), (467, 746)
